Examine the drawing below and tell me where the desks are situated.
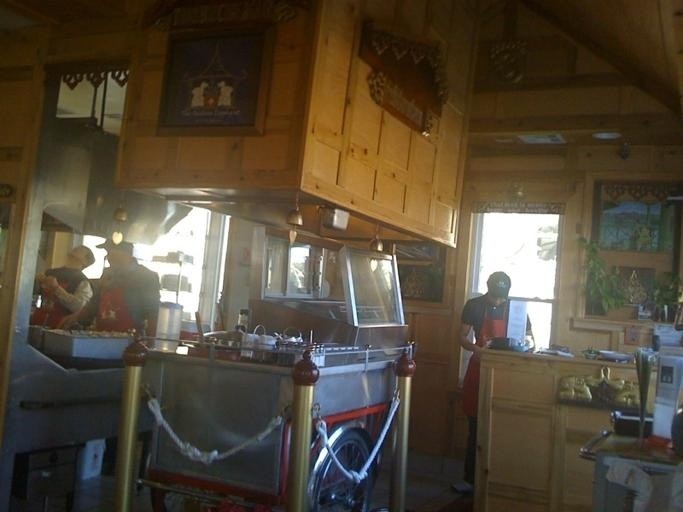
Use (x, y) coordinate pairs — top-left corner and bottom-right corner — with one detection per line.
(472, 345), (682, 510)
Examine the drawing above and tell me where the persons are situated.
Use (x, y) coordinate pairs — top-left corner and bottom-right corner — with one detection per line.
(452, 271), (534, 493)
(31, 245), (96, 327)
(59, 239), (161, 348)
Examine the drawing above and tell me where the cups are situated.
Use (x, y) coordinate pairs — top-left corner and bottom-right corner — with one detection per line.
(153, 302), (184, 353)
(240, 334), (276, 358)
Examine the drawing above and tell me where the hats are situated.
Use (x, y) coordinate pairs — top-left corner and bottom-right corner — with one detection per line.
(487, 272), (511, 298)
(96, 238), (133, 255)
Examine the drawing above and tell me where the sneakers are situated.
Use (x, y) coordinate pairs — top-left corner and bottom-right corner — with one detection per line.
(451, 480), (473, 494)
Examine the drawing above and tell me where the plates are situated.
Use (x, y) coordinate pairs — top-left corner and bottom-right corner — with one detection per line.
(599, 350), (634, 361)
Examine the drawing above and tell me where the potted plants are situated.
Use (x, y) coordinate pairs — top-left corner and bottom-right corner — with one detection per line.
(576, 236), (683, 325)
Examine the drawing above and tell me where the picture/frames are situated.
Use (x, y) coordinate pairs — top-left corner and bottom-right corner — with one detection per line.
(155, 18), (276, 136)
(589, 178), (682, 254)
